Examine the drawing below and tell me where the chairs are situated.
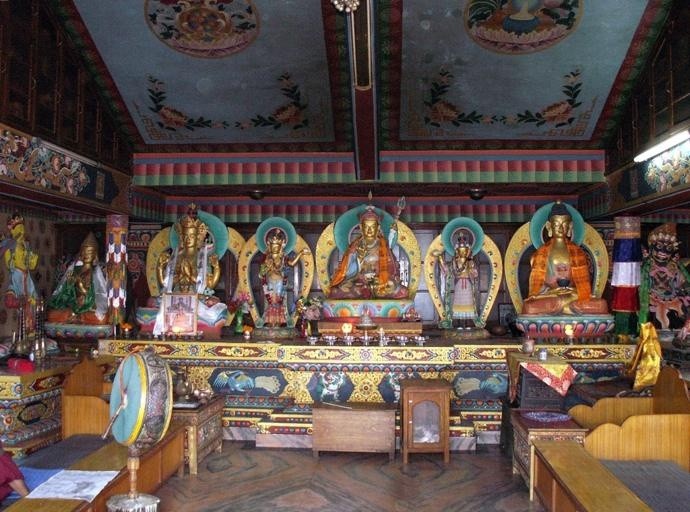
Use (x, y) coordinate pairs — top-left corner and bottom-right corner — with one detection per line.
(569, 364), (690, 474)
(61, 356), (118, 439)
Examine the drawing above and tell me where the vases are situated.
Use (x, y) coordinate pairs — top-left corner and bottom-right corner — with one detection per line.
(300, 321), (307, 337)
(235, 313), (243, 333)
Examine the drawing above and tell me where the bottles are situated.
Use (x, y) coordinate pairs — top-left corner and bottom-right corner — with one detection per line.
(538, 348), (547, 361)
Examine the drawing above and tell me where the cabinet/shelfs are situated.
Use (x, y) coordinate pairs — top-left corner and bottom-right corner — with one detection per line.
(312, 401), (396, 462)
(398, 377), (451, 466)
(510, 407), (590, 500)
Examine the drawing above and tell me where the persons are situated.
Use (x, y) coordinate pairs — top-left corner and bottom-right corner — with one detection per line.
(522, 200), (607, 314)
(332, 208), (409, 301)
(439, 230), (485, 331)
(642, 225), (690, 342)
(251, 226), (306, 328)
(156, 205), (224, 309)
(0, 209), (44, 315)
(0, 437), (29, 505)
(49, 234), (110, 315)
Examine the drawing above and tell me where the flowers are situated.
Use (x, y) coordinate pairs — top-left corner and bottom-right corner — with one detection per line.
(228, 290), (255, 314)
(296, 296), (322, 321)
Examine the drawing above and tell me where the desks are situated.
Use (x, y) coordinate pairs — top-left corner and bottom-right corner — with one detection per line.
(0, 420), (185, 512)
(497, 351), (572, 459)
(571, 380), (633, 407)
(169, 394), (226, 476)
(532, 439), (655, 512)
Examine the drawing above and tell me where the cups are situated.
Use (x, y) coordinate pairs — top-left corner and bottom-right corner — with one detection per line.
(522, 338), (535, 354)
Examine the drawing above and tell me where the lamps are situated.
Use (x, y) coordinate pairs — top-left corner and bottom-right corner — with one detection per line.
(468, 189), (487, 200)
(633, 128), (690, 164)
(247, 190), (265, 199)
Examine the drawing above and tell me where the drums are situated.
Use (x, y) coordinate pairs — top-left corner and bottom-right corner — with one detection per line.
(110, 350), (172, 451)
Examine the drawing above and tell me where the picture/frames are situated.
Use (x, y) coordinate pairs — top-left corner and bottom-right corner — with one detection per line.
(163, 292), (197, 337)
